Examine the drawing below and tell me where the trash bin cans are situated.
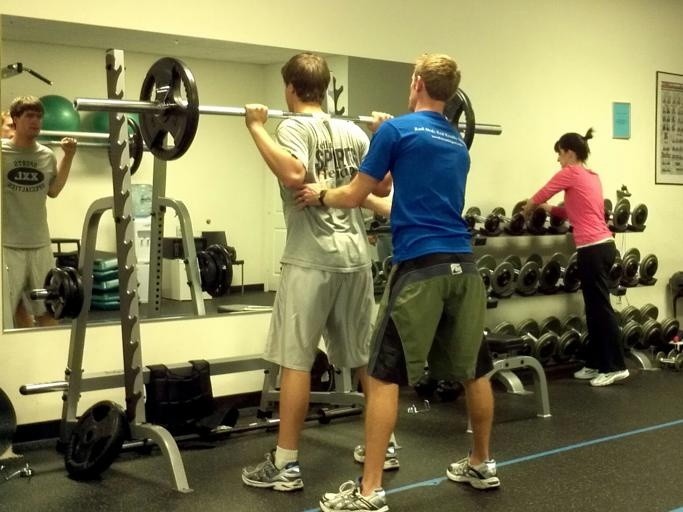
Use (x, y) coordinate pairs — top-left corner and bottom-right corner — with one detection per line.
(318, 188), (326, 206)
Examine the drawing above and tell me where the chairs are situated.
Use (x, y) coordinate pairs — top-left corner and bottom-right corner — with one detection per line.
(573, 366), (598, 379)
(353, 442), (400, 471)
(241, 447), (305, 492)
(446, 449), (500, 490)
(317, 475), (389, 512)
(590, 366), (630, 387)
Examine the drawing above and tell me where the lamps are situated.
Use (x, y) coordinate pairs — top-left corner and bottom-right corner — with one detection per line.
(201, 230), (245, 297)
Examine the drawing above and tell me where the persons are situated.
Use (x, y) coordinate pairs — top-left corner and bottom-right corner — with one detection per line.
(1, 111), (17, 139)
(519, 127), (630, 386)
(0, 96), (76, 332)
(291, 57), (500, 511)
(238, 51), (398, 492)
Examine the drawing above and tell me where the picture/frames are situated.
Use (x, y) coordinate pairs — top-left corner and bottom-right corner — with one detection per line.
(161, 256), (213, 302)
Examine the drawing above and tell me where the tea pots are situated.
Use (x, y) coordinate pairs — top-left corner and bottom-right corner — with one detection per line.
(0, 14), (417, 336)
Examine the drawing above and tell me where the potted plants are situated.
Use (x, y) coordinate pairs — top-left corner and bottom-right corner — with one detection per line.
(611, 101), (631, 139)
(655, 70), (683, 185)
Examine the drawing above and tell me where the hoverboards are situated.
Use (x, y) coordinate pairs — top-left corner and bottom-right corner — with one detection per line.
(0, 115), (175, 178)
(71, 56), (502, 159)
